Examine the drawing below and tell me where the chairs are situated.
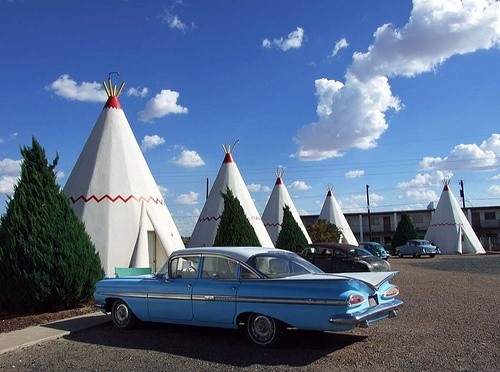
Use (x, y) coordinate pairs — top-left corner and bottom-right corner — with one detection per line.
(268, 259), (288, 275)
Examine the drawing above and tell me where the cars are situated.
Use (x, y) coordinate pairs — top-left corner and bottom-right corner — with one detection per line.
(92, 246), (404, 350)
(297, 242), (391, 272)
(396, 239), (442, 259)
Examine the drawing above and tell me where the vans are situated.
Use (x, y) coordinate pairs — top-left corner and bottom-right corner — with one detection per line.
(353, 242), (388, 261)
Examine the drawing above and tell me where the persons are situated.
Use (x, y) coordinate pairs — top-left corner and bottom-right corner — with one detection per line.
(431, 240), (435, 246)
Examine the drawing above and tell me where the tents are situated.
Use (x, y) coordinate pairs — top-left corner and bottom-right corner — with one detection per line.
(186, 141), (276, 250)
(423, 179), (486, 255)
(59, 80), (195, 281)
(315, 184), (360, 246)
(263, 172), (315, 255)
